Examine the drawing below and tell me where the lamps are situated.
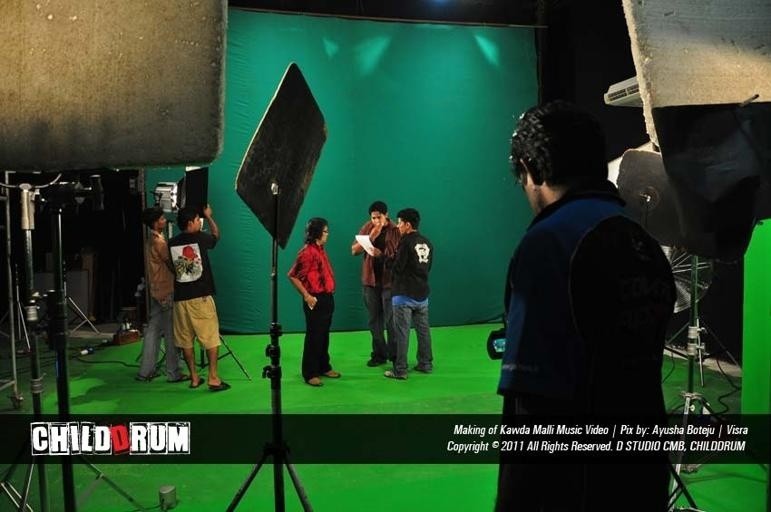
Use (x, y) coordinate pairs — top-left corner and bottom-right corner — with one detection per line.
(150, 166), (211, 224)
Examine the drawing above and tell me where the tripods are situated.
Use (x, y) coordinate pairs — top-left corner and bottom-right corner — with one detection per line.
(666, 255), (768, 511)
(0, 168), (145, 512)
(226, 182), (313, 511)
(151, 214), (252, 381)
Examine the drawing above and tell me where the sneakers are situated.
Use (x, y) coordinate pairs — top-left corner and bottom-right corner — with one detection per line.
(303, 367), (342, 387)
(366, 356), (390, 366)
(383, 368), (410, 380)
(413, 364), (432, 374)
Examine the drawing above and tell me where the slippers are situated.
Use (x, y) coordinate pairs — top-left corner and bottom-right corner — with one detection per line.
(131, 368), (233, 391)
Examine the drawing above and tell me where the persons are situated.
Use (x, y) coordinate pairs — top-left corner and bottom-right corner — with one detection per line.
(350, 200), (401, 366)
(167, 202), (231, 391)
(289, 216), (343, 385)
(496, 97), (677, 511)
(134, 206), (192, 383)
(370, 207), (433, 380)
